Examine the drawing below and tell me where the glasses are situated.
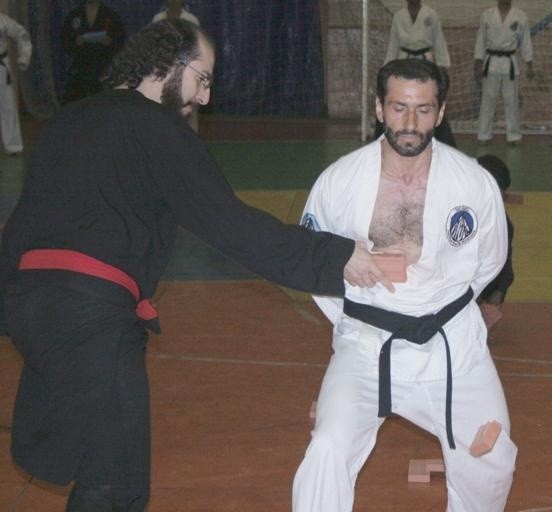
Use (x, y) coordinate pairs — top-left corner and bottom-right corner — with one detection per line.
(190, 61), (214, 89)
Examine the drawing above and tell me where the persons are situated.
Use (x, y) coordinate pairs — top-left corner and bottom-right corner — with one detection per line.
(1, 15), (396, 511)
(291, 58), (521, 512)
(1, 11), (34, 154)
(60, 0), (126, 105)
(473, 0), (535, 147)
(476, 153), (515, 317)
(375, 0), (458, 149)
(152, 1), (200, 133)
